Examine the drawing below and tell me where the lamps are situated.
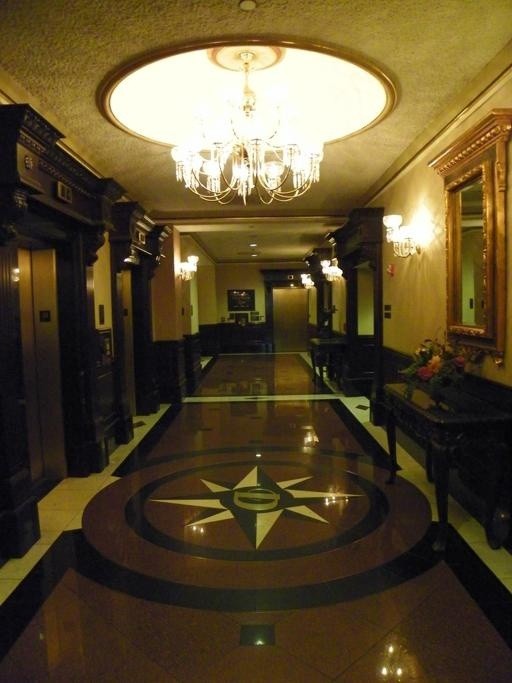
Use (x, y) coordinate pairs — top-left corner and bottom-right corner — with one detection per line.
(299, 271), (314, 289)
(381, 638), (418, 678)
(322, 484), (349, 509)
(383, 212), (422, 257)
(171, 46), (324, 204)
(179, 254), (200, 283)
(187, 514), (205, 536)
(317, 234), (343, 281)
(300, 424), (318, 452)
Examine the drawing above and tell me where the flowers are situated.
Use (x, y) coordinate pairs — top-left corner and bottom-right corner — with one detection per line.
(400, 330), (476, 411)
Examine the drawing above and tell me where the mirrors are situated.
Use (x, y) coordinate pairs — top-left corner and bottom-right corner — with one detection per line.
(445, 159), (493, 338)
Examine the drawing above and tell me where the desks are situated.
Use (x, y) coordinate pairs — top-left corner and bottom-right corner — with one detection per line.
(385, 380), (512, 536)
